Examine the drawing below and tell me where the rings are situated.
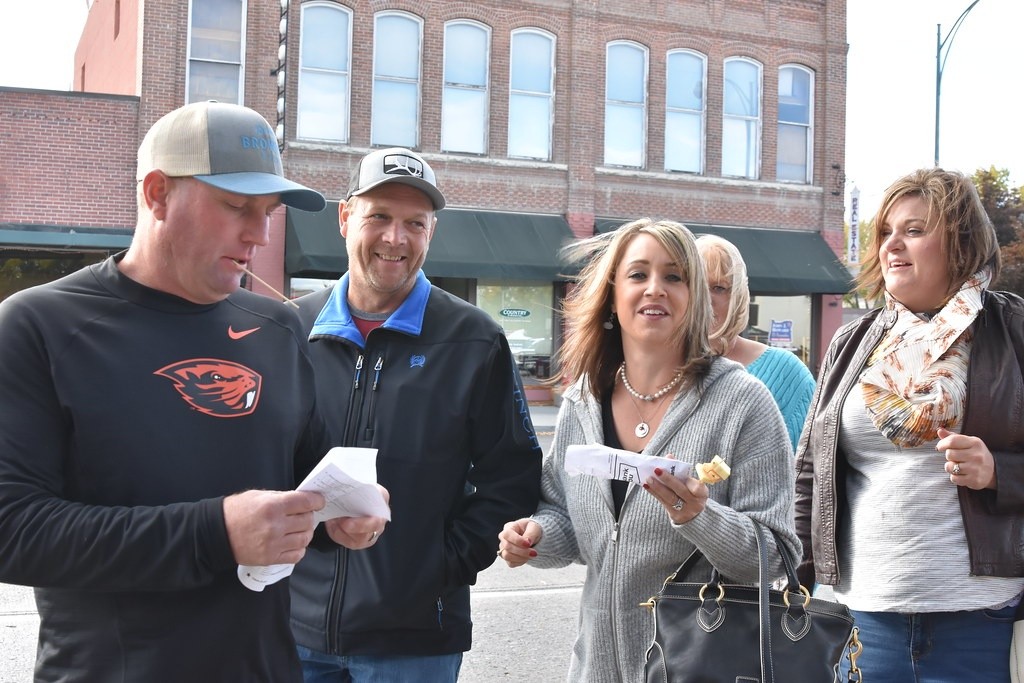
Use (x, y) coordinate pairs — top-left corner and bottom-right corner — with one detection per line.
(672, 499), (684, 510)
(369, 532), (377, 542)
(496, 548), (504, 559)
(952, 463), (961, 474)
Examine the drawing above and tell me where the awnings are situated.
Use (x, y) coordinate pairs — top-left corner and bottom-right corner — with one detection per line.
(592, 218), (858, 295)
(281, 196), (582, 283)
(0, 228), (133, 251)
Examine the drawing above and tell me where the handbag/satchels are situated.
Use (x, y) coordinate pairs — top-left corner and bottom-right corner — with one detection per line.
(639, 516), (862, 683)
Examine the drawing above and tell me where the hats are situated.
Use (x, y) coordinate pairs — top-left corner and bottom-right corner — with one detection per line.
(345, 148), (447, 210)
(136, 100), (327, 213)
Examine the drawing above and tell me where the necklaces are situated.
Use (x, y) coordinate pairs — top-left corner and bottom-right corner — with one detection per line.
(627, 390), (673, 437)
(621, 362), (678, 399)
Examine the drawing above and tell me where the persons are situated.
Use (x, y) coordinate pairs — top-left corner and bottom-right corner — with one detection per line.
(283, 148), (544, 683)
(493, 215), (825, 683)
(790, 172), (1024, 683)
(0, 100), (390, 683)
